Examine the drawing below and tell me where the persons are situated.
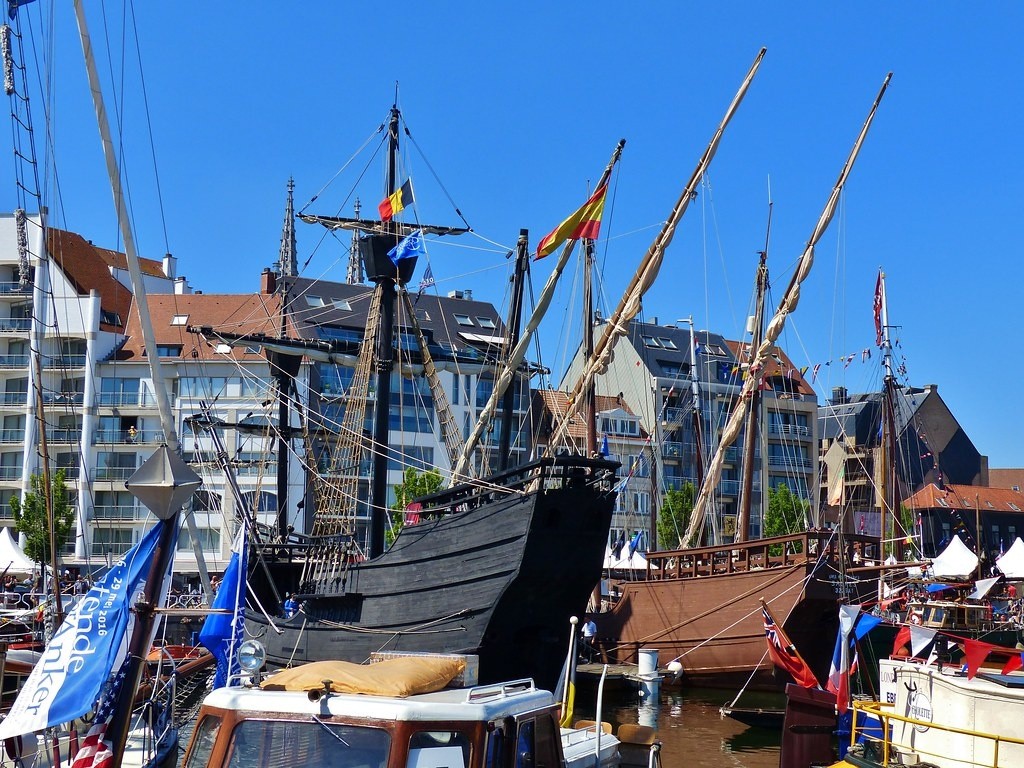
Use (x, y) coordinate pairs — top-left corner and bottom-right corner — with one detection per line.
(285, 593), (302, 619)
(1, 572), (88, 608)
(582, 615), (598, 665)
(210, 574), (221, 596)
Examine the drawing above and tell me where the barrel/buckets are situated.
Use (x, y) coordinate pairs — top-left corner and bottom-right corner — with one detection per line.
(639, 649), (659, 677)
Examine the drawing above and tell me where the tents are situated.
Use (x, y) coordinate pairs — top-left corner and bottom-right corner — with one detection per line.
(602, 542), (657, 570)
(932, 535), (979, 581)
(995, 537), (1024, 578)
(0, 526), (53, 573)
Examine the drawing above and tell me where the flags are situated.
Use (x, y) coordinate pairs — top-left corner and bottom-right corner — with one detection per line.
(872, 269), (883, 346)
(760, 599), (820, 688)
(67, 655), (129, 768)
(378, 178), (418, 222)
(198, 521), (250, 691)
(1, 516), (173, 736)
(386, 229), (427, 266)
(825, 604), (857, 716)
(535, 183), (607, 261)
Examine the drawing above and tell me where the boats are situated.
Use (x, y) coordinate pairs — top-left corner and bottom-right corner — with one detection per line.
(0, 1), (1024, 768)
